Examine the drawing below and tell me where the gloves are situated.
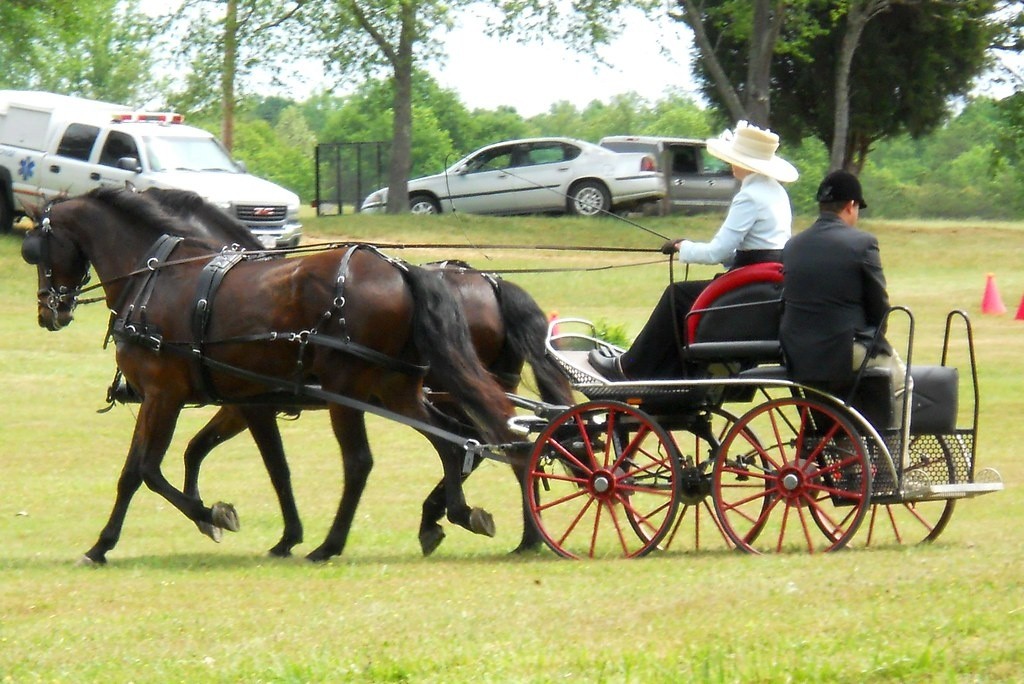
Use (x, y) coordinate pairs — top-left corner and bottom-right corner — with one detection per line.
(661, 238), (684, 254)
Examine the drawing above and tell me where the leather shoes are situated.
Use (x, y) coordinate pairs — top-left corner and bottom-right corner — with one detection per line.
(588, 347), (624, 382)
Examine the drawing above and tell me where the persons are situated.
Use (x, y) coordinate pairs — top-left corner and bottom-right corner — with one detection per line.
(589, 120), (799, 382)
(780, 170), (920, 489)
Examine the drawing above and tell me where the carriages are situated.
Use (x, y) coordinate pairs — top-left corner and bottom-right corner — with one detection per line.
(17, 179), (1004, 570)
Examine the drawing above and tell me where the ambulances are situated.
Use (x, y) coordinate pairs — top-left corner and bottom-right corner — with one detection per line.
(1, 88), (303, 256)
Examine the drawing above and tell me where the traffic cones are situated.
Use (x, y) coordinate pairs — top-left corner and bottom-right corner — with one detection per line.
(983, 272), (1007, 316)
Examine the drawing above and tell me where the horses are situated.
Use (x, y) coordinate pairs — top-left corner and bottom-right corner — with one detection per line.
(19, 177), (641, 573)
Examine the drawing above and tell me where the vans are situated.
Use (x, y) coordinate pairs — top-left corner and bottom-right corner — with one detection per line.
(600, 135), (742, 215)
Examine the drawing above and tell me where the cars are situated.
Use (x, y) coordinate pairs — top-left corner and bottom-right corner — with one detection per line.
(358, 136), (666, 217)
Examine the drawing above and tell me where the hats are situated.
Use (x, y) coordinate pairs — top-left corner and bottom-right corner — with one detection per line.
(818, 169), (867, 210)
(705, 120), (800, 184)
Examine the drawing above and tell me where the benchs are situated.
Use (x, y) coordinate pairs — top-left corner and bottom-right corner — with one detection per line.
(877, 364), (959, 435)
(681, 250), (783, 364)
(738, 366), (899, 431)
